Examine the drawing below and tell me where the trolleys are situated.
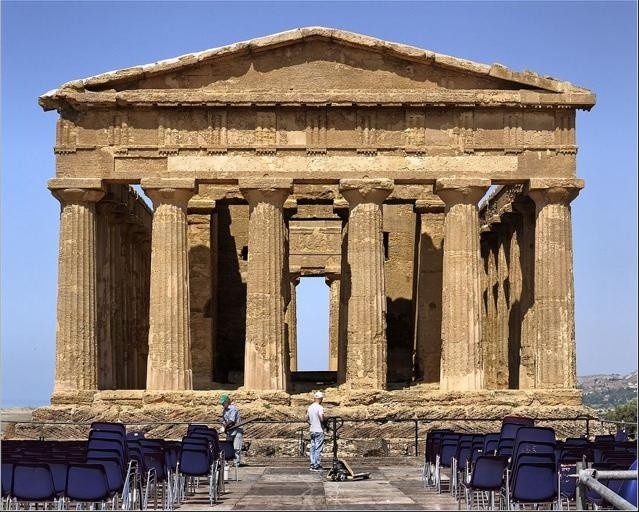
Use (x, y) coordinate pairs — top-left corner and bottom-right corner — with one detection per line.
(324, 415), (370, 481)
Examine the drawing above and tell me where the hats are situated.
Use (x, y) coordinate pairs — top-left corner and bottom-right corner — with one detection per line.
(219, 395), (229, 405)
(314, 391), (324, 399)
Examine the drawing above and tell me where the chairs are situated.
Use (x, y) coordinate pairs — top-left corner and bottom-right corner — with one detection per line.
(1, 422), (238, 511)
(424, 417), (638, 511)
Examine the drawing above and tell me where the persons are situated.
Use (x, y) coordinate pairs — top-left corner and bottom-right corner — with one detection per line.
(304, 392), (329, 472)
(218, 394), (244, 468)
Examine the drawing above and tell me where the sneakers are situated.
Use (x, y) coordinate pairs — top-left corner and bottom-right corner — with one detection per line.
(309, 464), (327, 472)
